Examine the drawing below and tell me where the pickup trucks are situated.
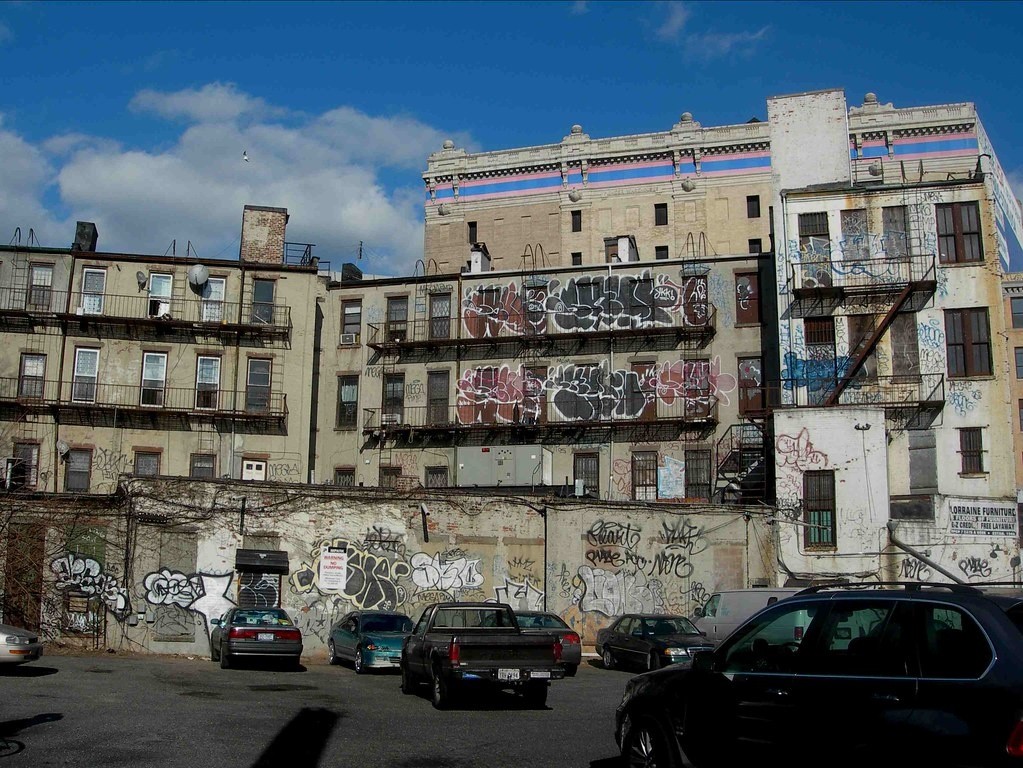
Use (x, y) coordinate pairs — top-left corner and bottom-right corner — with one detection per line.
(399, 601), (581, 709)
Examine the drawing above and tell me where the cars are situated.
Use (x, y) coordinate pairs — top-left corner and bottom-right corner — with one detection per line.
(0, 624), (43, 665)
(327, 611), (414, 674)
(209, 606), (304, 670)
(595, 614), (714, 669)
(472, 610), (581, 677)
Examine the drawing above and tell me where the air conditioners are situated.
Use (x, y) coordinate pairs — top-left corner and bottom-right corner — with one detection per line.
(340, 333), (357, 345)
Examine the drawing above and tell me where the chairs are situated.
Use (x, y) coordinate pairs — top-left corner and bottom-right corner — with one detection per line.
(235, 616), (247, 622)
(934, 628), (968, 656)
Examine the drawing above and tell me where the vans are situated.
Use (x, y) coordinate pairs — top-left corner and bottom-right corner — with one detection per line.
(690, 587), (853, 651)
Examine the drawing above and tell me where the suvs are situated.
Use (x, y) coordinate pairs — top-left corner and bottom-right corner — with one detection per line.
(613, 581), (1023, 768)
